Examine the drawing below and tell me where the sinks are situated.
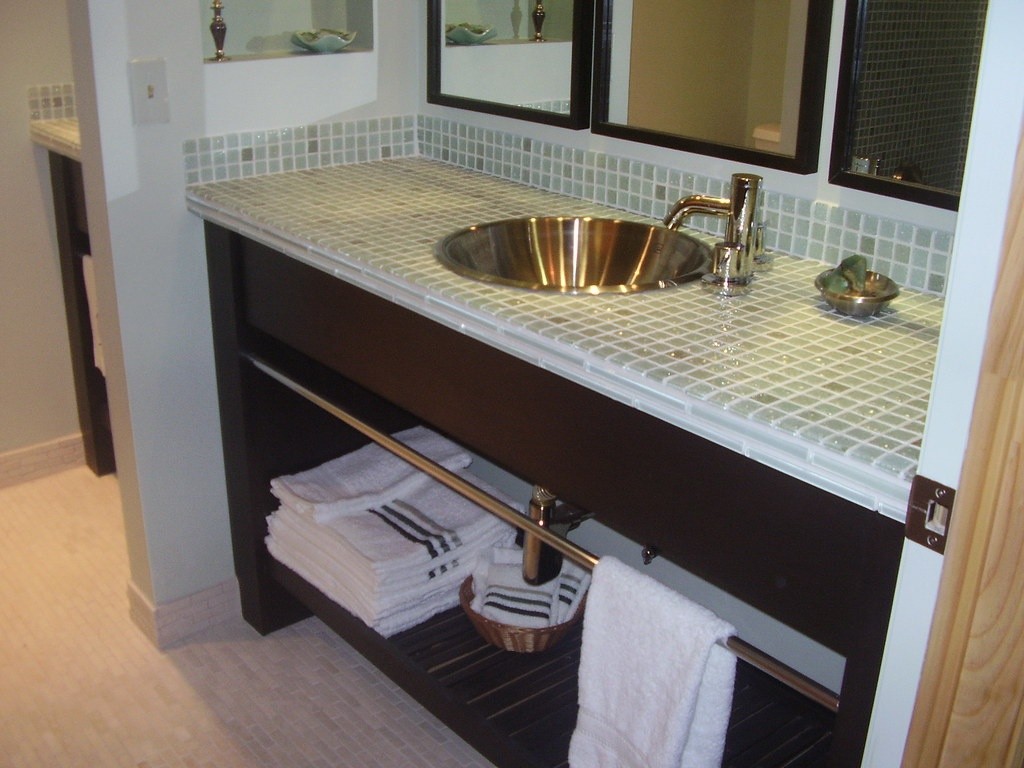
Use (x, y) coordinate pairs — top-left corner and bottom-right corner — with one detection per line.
(431, 213), (716, 297)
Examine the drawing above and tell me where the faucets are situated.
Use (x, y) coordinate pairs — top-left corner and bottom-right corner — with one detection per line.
(661, 172), (775, 298)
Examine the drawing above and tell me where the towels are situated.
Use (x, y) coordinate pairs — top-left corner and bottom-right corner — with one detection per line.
(262, 423), (593, 641)
(566, 554), (740, 768)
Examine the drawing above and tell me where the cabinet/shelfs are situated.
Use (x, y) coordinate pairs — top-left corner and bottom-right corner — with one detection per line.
(43, 150), (112, 479)
(201, 219), (908, 768)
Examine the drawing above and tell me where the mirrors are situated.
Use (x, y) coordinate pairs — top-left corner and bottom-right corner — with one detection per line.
(827, 0), (988, 213)
(427, 0), (596, 131)
(590, 0), (835, 177)
